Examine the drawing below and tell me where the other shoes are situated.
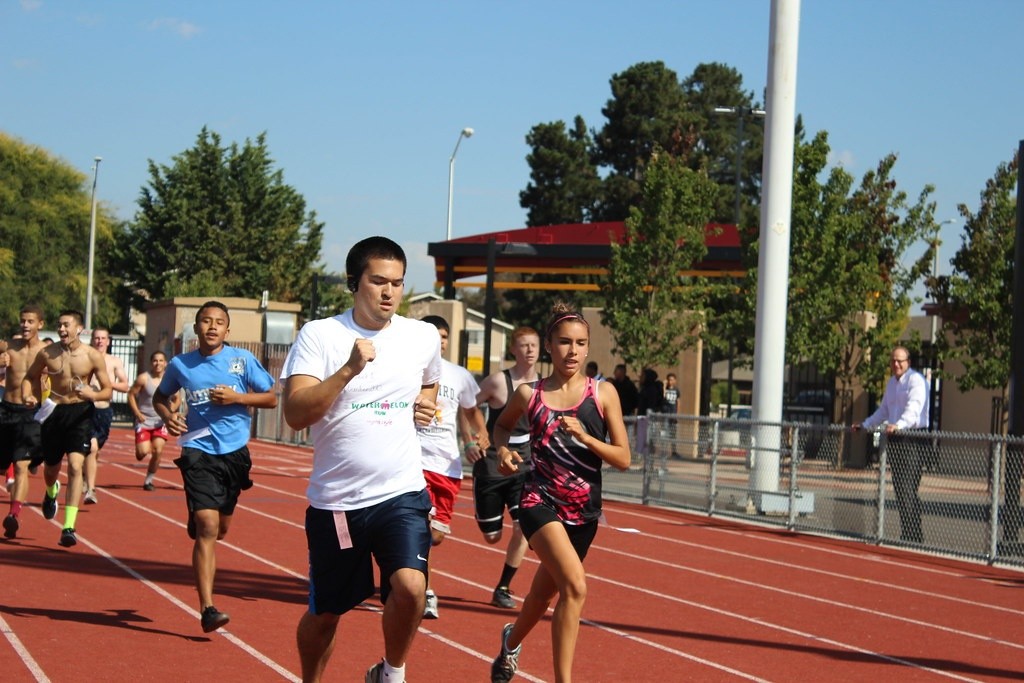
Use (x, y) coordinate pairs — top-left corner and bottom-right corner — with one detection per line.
(82, 480), (88, 493)
(84, 488), (97, 504)
(3, 513), (18, 538)
(671, 452), (682, 459)
(143, 482), (155, 491)
(29, 467), (38, 475)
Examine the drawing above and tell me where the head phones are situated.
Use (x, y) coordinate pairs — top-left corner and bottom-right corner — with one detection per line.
(347, 278), (358, 293)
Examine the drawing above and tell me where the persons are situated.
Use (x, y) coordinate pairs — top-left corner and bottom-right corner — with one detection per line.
(279, 236), (442, 683)
(128, 351), (181, 491)
(586, 362), (681, 471)
(0, 306), (129, 547)
(460, 327), (544, 608)
(379, 315), (491, 618)
(492, 302), (630, 683)
(851, 347), (930, 542)
(152, 301), (278, 634)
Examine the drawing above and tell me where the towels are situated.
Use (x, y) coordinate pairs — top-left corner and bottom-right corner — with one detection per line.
(32, 396), (57, 425)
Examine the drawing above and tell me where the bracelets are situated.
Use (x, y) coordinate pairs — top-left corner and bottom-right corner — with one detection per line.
(464, 442), (474, 451)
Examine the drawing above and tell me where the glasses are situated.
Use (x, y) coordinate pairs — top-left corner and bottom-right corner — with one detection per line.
(890, 358), (909, 366)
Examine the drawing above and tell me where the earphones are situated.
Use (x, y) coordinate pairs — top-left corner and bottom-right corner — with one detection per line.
(77, 328), (80, 334)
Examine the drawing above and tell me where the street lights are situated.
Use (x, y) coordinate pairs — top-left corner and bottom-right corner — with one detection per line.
(85, 155), (102, 333)
(446, 126), (474, 241)
(925, 217), (957, 433)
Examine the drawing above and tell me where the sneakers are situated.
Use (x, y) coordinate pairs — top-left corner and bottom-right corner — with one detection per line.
(365, 657), (406, 683)
(491, 587), (517, 608)
(200, 606), (230, 633)
(60, 528), (77, 547)
(42, 479), (61, 521)
(186, 496), (197, 540)
(490, 623), (521, 683)
(423, 594), (438, 618)
